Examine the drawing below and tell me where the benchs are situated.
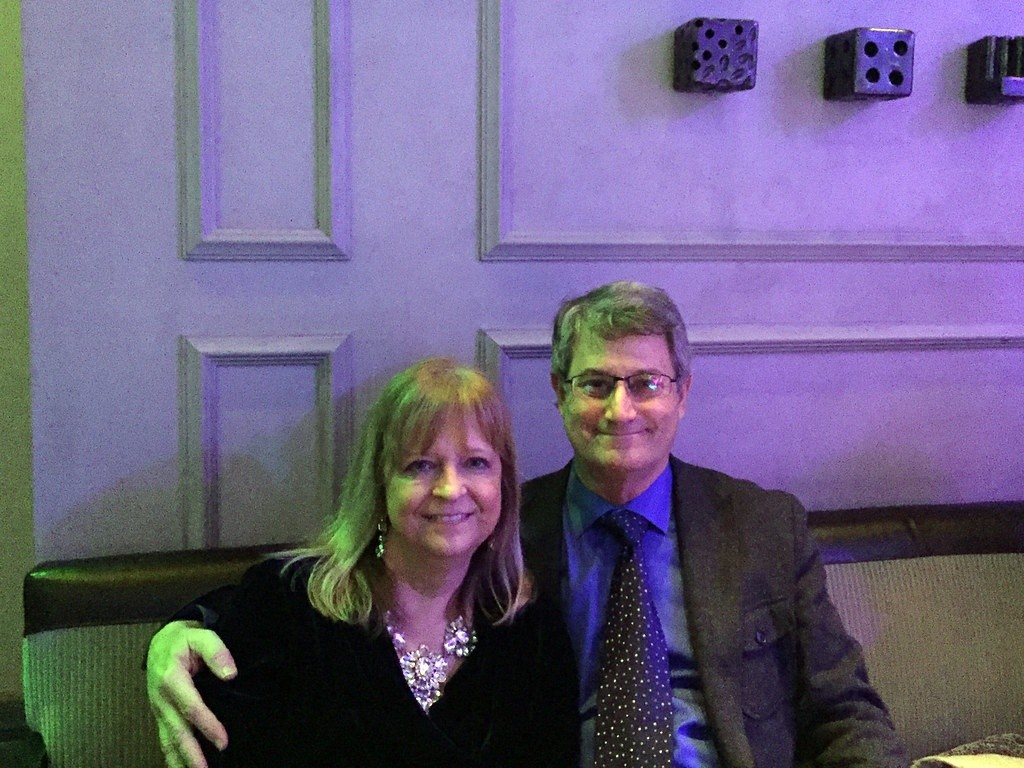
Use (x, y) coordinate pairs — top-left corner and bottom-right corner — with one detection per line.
(24, 497), (1024, 768)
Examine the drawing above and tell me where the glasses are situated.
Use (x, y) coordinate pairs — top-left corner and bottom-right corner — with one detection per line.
(565, 373), (678, 402)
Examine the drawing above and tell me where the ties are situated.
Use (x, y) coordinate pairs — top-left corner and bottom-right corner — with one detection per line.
(591, 508), (673, 768)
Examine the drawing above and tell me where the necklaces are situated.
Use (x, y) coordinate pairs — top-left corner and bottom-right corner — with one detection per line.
(381, 607), (480, 712)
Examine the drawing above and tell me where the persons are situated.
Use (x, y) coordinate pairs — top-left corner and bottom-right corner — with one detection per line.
(192, 357), (591, 768)
(139, 280), (913, 767)
